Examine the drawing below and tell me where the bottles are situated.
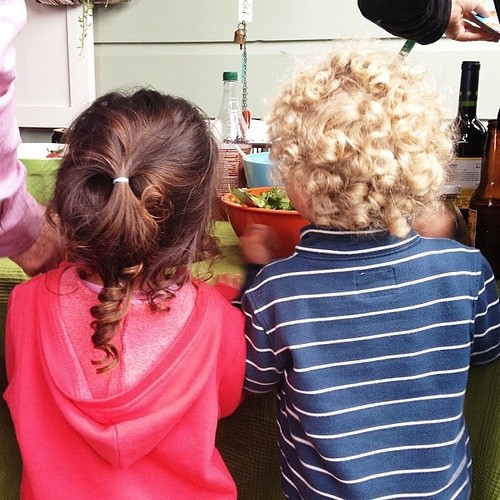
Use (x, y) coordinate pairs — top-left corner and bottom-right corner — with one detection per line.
(210, 71), (252, 197)
(446, 61), (486, 221)
(468, 120), (500, 279)
(440, 185), (470, 246)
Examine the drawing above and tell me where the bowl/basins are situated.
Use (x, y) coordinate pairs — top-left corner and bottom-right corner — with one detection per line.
(243, 152), (275, 187)
(220, 187), (312, 258)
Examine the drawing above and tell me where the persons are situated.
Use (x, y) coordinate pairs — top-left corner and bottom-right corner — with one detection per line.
(3, 86), (247, 500)
(0, 0), (66, 278)
(230, 34), (500, 500)
